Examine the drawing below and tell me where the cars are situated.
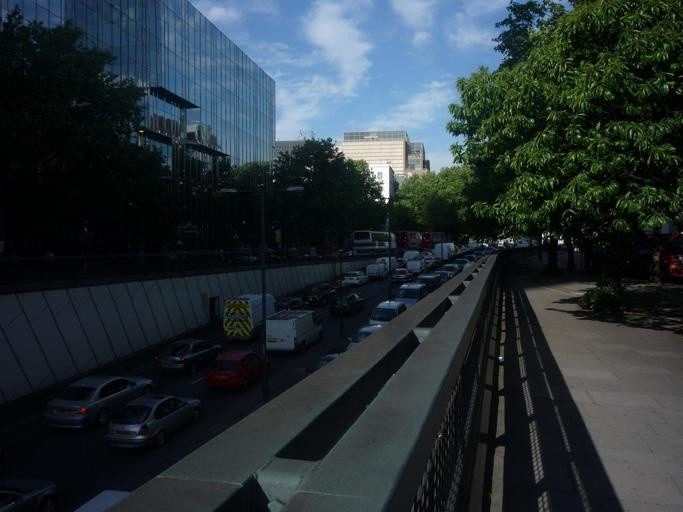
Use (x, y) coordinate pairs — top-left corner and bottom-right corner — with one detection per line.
(205, 348), (265, 391)
(266, 229), (532, 368)
(43, 373), (154, 432)
(103, 392), (201, 448)
(154, 338), (222, 374)
(0, 477), (56, 512)
(215, 248), (259, 269)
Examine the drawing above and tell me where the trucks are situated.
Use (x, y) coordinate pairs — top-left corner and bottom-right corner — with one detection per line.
(222, 291), (278, 344)
